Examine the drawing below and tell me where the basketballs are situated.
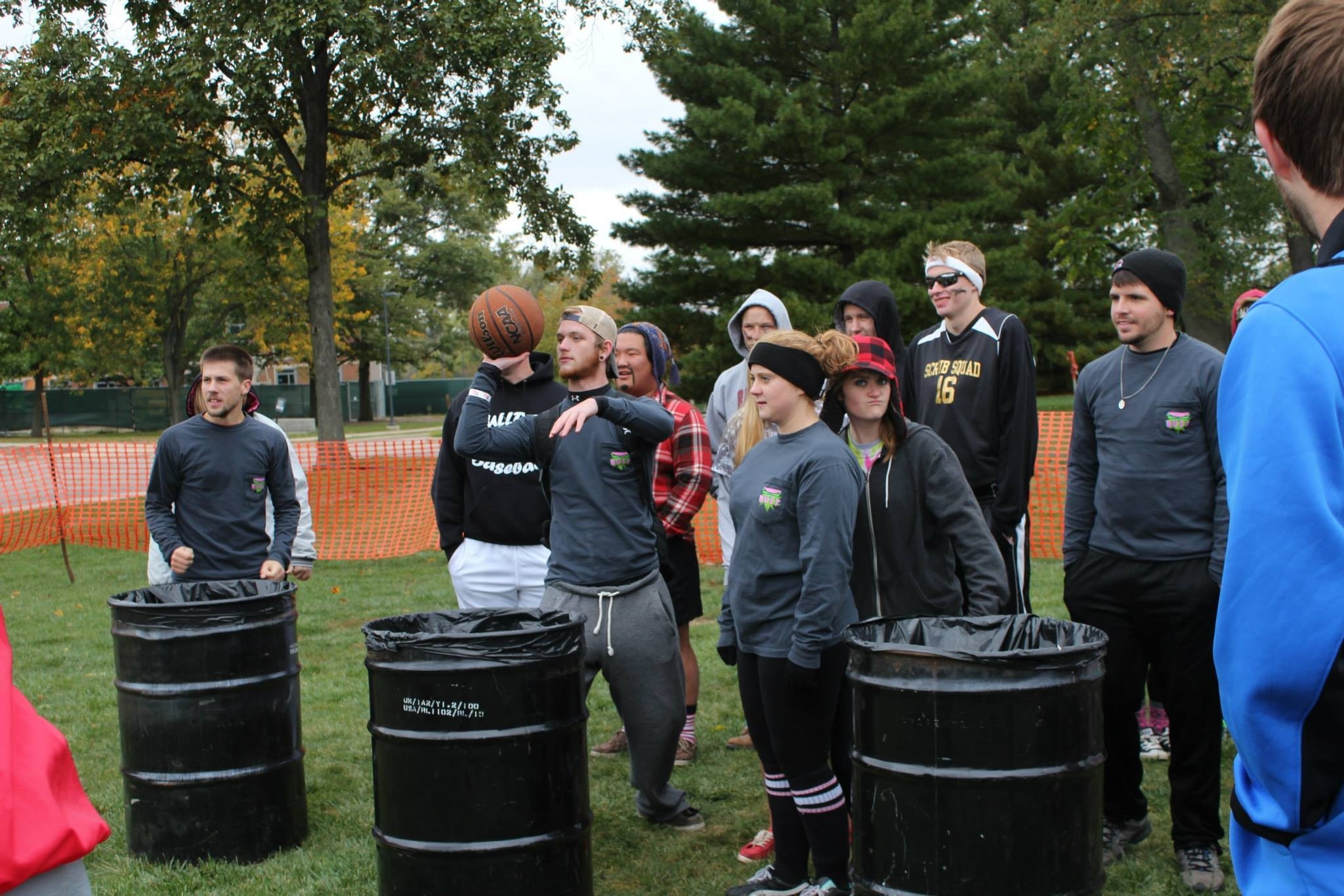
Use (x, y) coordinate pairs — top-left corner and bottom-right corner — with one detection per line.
(468, 285), (544, 359)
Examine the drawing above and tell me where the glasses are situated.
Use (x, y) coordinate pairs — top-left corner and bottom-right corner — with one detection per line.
(925, 271), (964, 289)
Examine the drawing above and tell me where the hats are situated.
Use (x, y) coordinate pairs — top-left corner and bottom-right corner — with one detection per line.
(1111, 248), (1186, 323)
(616, 321), (681, 405)
(819, 334), (908, 442)
(560, 305), (620, 379)
(1236, 302), (1254, 321)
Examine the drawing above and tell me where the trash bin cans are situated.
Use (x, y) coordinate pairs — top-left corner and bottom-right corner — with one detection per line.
(106, 582), (311, 862)
(846, 615), (1111, 896)
(363, 605), (587, 896)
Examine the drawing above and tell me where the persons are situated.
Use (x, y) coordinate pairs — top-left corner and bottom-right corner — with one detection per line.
(431, 240), (1268, 896)
(144, 345), (316, 585)
(1218, 0), (1344, 896)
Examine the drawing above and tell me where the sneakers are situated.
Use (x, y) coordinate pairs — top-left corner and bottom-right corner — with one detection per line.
(589, 730), (629, 756)
(1103, 815), (1152, 866)
(1178, 846), (1225, 893)
(736, 829), (776, 863)
(1138, 727), (1170, 761)
(674, 737), (697, 766)
(798, 877), (854, 896)
(723, 865), (812, 895)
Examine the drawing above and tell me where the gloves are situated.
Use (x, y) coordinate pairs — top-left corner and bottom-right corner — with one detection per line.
(716, 646), (737, 666)
(786, 659), (819, 685)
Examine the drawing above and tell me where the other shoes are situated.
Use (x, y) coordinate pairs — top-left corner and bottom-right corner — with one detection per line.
(661, 808), (706, 832)
(724, 726), (752, 748)
